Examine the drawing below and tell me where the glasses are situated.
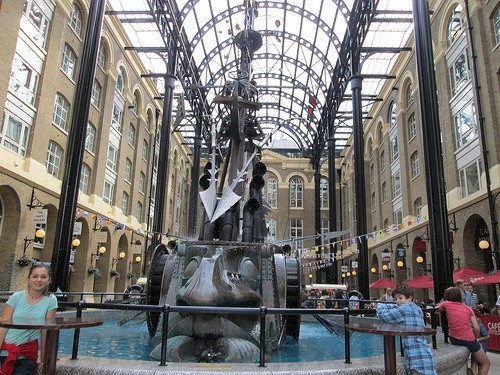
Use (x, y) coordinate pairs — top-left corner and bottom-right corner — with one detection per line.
(463, 284), (469, 286)
(31, 261), (51, 267)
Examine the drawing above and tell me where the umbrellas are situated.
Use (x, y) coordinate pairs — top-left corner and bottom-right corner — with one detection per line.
(405, 275), (434, 288)
(453, 266), (500, 286)
(370, 278), (400, 288)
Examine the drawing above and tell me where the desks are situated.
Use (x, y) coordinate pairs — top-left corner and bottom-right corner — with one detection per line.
(0, 318), (103, 375)
(345, 322), (438, 375)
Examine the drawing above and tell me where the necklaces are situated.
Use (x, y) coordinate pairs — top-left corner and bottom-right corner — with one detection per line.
(27, 290), (43, 300)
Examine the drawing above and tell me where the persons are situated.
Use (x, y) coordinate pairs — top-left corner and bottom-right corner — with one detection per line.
(377, 284), (437, 375)
(0, 263), (58, 375)
(300, 288), (394, 318)
(434, 285), (491, 375)
(456, 280), (500, 354)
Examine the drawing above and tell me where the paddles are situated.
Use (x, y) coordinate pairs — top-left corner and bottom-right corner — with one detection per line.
(138, 318), (147, 325)
(117, 311), (144, 326)
(118, 297), (146, 304)
(303, 306), (354, 337)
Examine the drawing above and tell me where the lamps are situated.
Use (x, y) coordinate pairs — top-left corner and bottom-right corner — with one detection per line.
(480, 239), (500, 266)
(397, 260), (410, 276)
(130, 231), (142, 246)
(91, 247), (106, 264)
(129, 256), (141, 271)
(94, 218), (108, 232)
(416, 256), (431, 273)
(383, 264), (394, 277)
(112, 252), (125, 268)
(370, 268), (381, 279)
(71, 239), (81, 251)
(27, 188), (51, 210)
(342, 271), (356, 278)
(24, 229), (46, 253)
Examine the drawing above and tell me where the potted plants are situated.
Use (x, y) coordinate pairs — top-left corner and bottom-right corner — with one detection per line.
(110, 270), (121, 279)
(127, 273), (136, 280)
(17, 258), (36, 266)
(88, 267), (103, 279)
